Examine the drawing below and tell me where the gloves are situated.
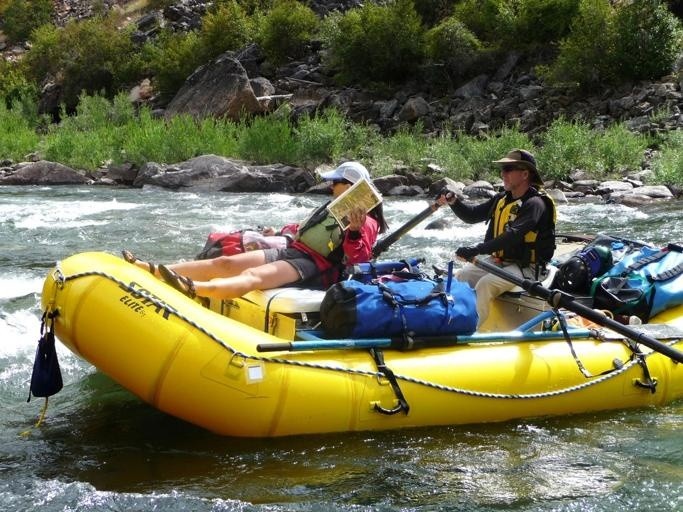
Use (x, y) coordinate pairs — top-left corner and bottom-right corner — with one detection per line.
(456, 246), (479, 263)
(435, 189), (456, 205)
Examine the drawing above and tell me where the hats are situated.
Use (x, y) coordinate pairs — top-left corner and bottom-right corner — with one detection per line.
(490, 148), (543, 186)
(320, 161), (371, 187)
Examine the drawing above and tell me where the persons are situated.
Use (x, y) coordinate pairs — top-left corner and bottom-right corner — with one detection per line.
(435, 148), (556, 328)
(121, 162), (386, 300)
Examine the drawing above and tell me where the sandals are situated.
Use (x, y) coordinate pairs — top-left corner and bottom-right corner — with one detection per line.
(122, 249), (155, 276)
(157, 263), (195, 299)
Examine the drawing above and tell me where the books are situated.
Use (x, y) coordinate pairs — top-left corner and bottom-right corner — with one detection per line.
(326, 176), (382, 231)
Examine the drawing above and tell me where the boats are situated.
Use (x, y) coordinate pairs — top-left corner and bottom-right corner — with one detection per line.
(41, 242), (683, 438)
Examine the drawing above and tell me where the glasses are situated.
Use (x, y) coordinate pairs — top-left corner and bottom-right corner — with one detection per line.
(332, 178), (351, 187)
(499, 165), (515, 173)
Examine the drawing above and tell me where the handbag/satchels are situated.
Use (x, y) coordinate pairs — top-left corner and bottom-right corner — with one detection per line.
(591, 244), (683, 324)
(320, 276), (479, 339)
(553, 246), (613, 296)
